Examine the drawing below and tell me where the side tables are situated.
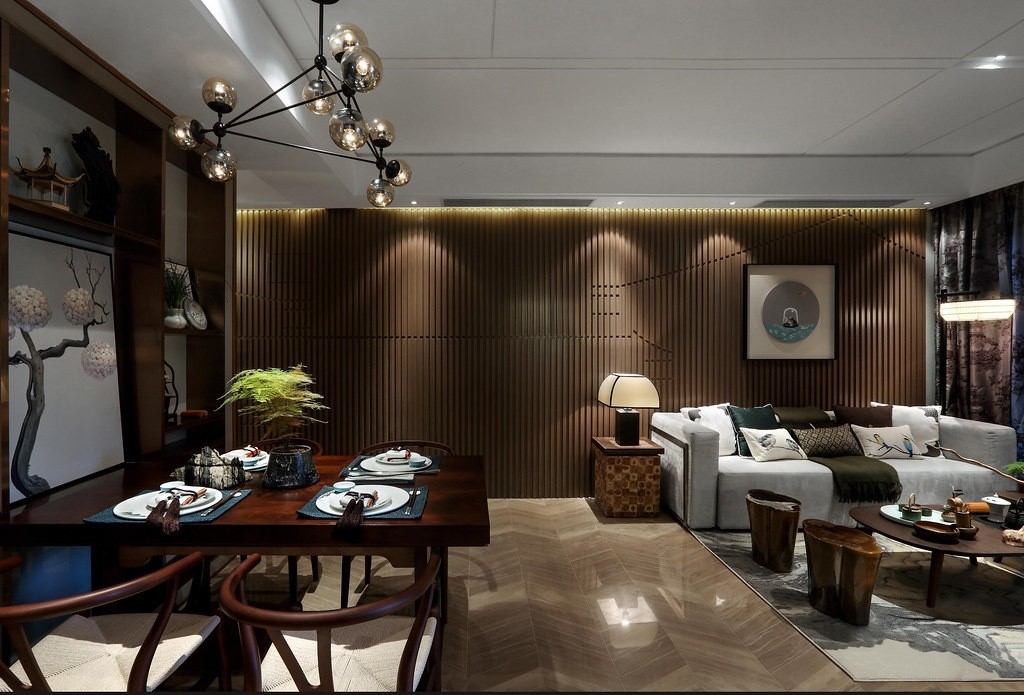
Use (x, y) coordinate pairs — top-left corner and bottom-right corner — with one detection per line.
(592, 436), (665, 517)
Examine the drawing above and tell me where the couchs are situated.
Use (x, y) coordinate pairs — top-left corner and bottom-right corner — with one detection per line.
(648, 401), (1018, 530)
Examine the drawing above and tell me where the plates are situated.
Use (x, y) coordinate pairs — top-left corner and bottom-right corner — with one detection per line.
(315, 484), (410, 516)
(360, 455), (433, 472)
(147, 486), (215, 509)
(329, 490), (391, 511)
(220, 451), (271, 470)
(112, 485), (223, 520)
(374, 452), (421, 464)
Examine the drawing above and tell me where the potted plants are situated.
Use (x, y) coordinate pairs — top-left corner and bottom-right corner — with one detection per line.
(213, 364), (331, 488)
(1003, 461), (1024, 493)
(164, 258), (192, 329)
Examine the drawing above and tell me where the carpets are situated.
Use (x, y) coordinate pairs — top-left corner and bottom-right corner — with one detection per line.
(688, 528), (1023, 681)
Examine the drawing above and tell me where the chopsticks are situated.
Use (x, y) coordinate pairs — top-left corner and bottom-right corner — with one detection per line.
(404, 486), (418, 514)
(200, 488), (241, 516)
(352, 457), (366, 469)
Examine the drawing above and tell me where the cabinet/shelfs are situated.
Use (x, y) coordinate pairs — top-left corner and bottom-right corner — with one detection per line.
(165, 133), (225, 450)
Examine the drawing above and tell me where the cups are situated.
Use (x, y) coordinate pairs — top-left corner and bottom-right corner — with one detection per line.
(982, 492), (1012, 523)
(899, 504), (906, 512)
(921, 508), (932, 516)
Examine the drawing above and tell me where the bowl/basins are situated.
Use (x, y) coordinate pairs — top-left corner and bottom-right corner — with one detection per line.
(333, 480), (355, 492)
(409, 457), (426, 467)
(160, 481), (185, 491)
(232, 449), (268, 466)
(913, 520), (960, 542)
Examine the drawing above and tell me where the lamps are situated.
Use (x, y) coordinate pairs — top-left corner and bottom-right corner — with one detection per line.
(597, 372), (660, 446)
(168, 0), (413, 208)
(937, 287), (1017, 416)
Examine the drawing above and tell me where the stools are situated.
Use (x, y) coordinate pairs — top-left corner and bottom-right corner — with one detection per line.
(745, 489), (802, 574)
(803, 517), (882, 628)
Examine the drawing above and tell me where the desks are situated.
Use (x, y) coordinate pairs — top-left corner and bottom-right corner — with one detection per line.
(0, 454), (489, 669)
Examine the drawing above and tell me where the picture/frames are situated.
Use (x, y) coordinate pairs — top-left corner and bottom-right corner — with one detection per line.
(744, 263), (839, 361)
(9, 229), (128, 506)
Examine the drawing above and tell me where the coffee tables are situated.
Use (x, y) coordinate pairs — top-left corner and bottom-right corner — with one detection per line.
(850, 501), (1024, 606)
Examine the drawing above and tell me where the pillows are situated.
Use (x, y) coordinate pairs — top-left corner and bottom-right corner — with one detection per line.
(680, 402), (943, 461)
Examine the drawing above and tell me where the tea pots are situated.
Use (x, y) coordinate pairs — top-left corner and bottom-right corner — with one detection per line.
(902, 493), (922, 521)
(1005, 497), (1024, 530)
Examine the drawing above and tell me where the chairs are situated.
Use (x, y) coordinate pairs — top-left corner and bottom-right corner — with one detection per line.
(342, 441), (454, 621)
(219, 552), (442, 693)
(202, 438), (323, 602)
(0, 551), (233, 692)
